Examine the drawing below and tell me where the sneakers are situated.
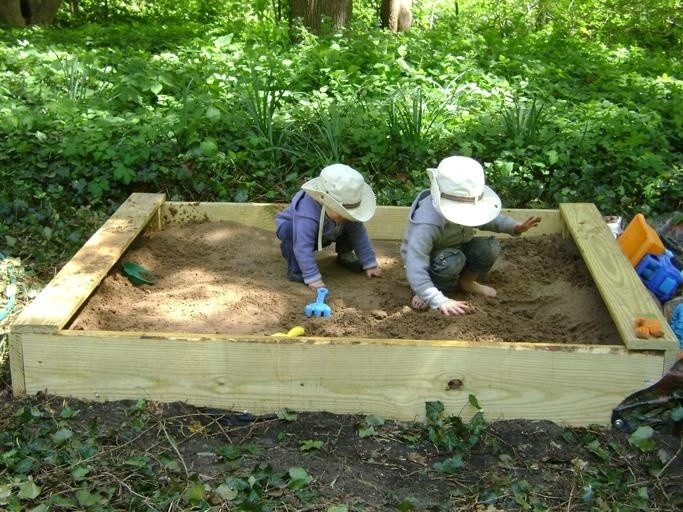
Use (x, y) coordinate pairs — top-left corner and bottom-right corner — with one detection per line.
(287, 269), (304, 282)
(337, 253), (364, 273)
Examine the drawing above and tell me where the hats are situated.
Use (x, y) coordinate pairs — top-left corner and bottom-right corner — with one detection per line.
(426, 156), (502, 227)
(301, 163), (376, 223)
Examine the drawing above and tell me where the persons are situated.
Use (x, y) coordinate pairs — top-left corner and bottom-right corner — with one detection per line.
(399, 156), (541, 316)
(274, 164), (382, 292)
